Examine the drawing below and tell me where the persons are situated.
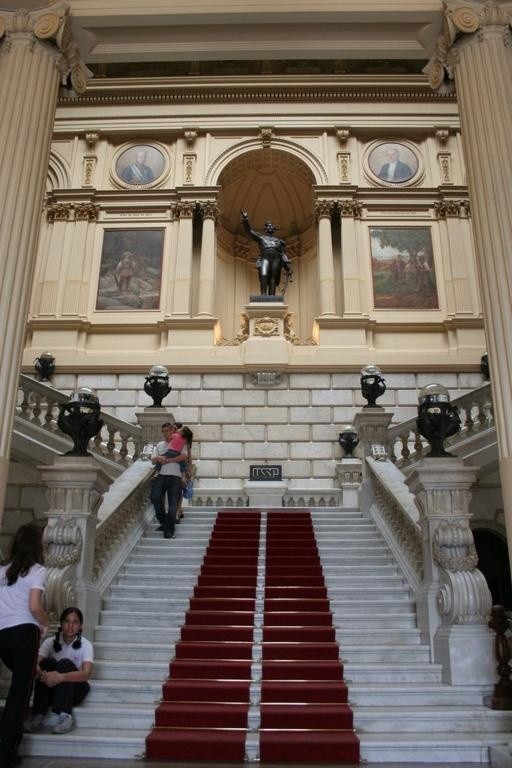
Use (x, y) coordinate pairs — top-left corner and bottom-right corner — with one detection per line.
(239, 206), (287, 295)
(120, 149), (156, 185)
(114, 251), (138, 291)
(0, 523), (94, 767)
(149, 422), (193, 538)
(377, 148), (413, 182)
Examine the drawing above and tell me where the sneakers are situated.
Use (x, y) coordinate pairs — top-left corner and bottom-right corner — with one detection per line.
(51, 717), (76, 734)
(155, 510), (184, 538)
(23, 717), (44, 733)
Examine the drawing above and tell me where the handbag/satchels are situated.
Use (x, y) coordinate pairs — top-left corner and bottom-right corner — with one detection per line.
(183, 480), (193, 499)
